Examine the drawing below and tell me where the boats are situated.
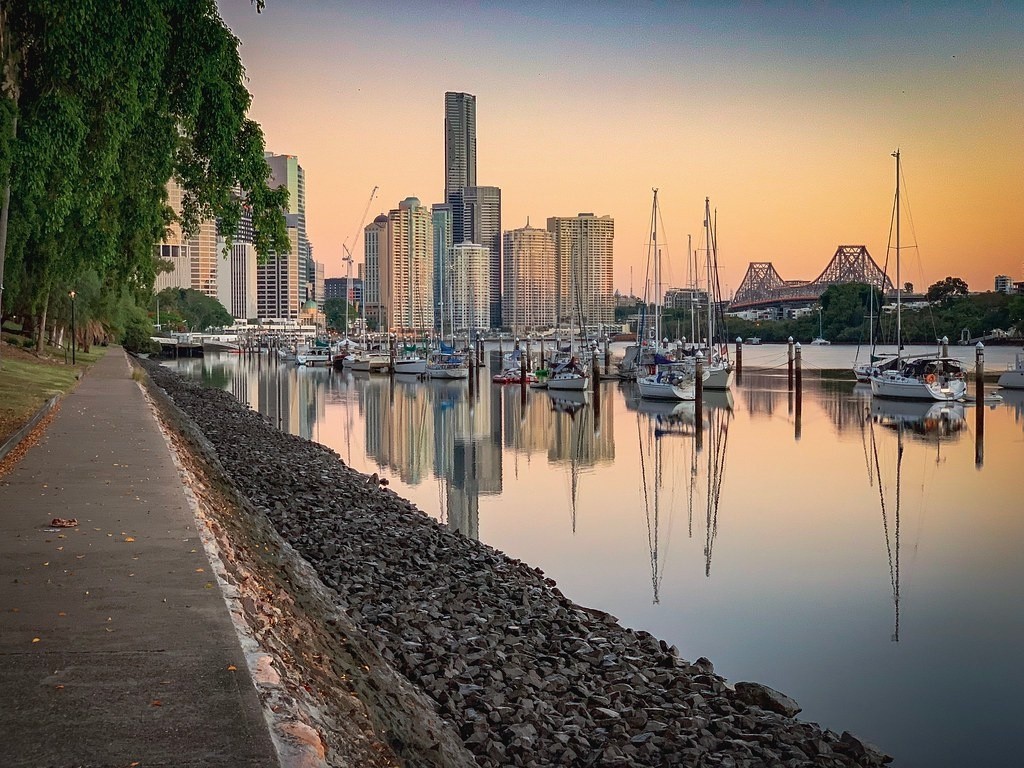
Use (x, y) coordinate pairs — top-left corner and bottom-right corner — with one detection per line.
(744, 337), (762, 345)
(962, 392), (1003, 402)
(998, 348), (1024, 388)
(957, 328), (1008, 346)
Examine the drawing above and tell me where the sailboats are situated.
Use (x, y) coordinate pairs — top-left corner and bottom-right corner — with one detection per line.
(636, 398), (695, 605)
(865, 399), (967, 642)
(867, 147), (967, 400)
(811, 306), (830, 346)
(702, 390), (735, 577)
(547, 390), (589, 533)
(277, 187), (734, 400)
(852, 273), (882, 383)
(853, 381), (874, 487)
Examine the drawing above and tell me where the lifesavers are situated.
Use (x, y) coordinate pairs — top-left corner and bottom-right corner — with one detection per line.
(926, 373), (936, 384)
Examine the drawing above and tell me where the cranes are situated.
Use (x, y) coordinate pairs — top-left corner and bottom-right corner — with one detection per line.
(341, 185), (379, 334)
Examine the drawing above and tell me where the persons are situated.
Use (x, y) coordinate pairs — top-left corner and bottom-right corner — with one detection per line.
(657, 364), (683, 386)
(692, 346), (696, 356)
